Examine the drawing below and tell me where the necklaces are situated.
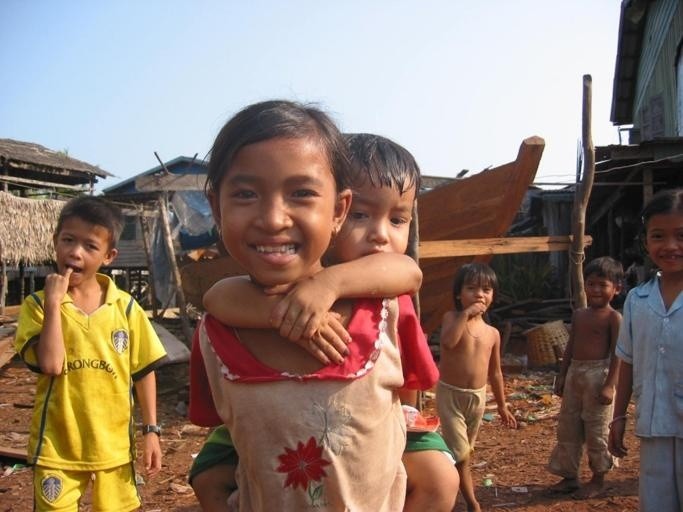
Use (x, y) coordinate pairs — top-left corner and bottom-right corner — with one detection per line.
(465, 322), (490, 338)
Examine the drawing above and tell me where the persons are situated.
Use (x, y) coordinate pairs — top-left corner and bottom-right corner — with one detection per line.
(16, 194), (169, 511)
(186, 133), (461, 511)
(543, 255), (623, 498)
(605, 188), (683, 511)
(435, 262), (517, 511)
(187, 99), (440, 512)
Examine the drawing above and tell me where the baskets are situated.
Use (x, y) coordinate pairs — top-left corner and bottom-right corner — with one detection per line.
(522, 319), (569, 370)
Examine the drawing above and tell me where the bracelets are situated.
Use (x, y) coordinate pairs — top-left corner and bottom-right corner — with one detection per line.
(142, 424), (162, 437)
(607, 415), (626, 430)
